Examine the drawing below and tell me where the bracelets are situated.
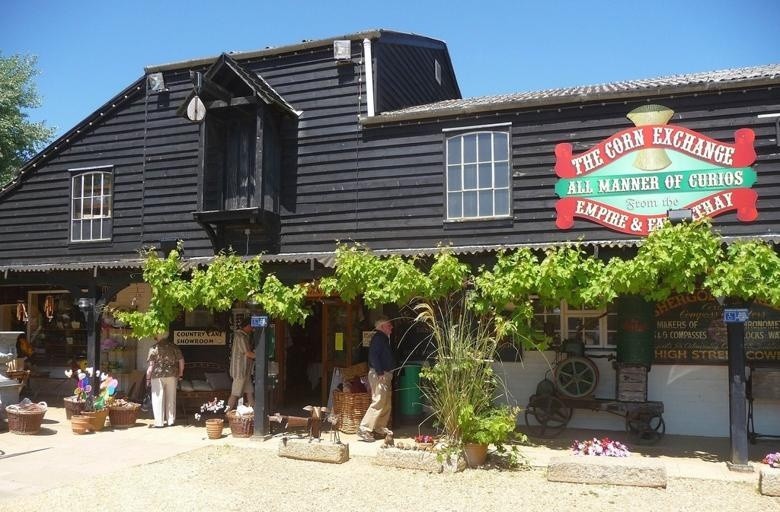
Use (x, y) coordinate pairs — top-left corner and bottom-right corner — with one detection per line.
(179, 375), (184, 378)
(146, 378), (151, 381)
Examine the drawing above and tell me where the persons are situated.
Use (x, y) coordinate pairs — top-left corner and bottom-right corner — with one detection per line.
(354, 314), (397, 445)
(145, 325), (185, 428)
(223, 317), (256, 419)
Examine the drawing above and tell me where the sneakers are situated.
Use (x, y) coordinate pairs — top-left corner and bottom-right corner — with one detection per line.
(373, 430), (394, 439)
(357, 429), (375, 442)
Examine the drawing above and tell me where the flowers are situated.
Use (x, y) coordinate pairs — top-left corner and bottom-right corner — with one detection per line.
(571, 438), (629, 458)
(195, 397), (230, 421)
(761, 452), (780, 468)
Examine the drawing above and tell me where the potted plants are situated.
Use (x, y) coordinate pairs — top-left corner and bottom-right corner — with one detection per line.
(356, 287), (555, 473)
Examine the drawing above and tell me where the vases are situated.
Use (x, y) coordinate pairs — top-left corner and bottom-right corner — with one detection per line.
(70, 410), (110, 434)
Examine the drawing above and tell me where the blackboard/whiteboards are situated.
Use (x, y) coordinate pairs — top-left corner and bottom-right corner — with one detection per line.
(649, 281), (780, 368)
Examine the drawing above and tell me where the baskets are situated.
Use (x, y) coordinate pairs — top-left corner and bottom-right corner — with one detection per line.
(351, 362), (367, 377)
(227, 410), (254, 438)
(72, 408), (108, 434)
(108, 404), (141, 428)
(63, 395), (86, 420)
(332, 388), (372, 434)
(5, 401), (48, 434)
